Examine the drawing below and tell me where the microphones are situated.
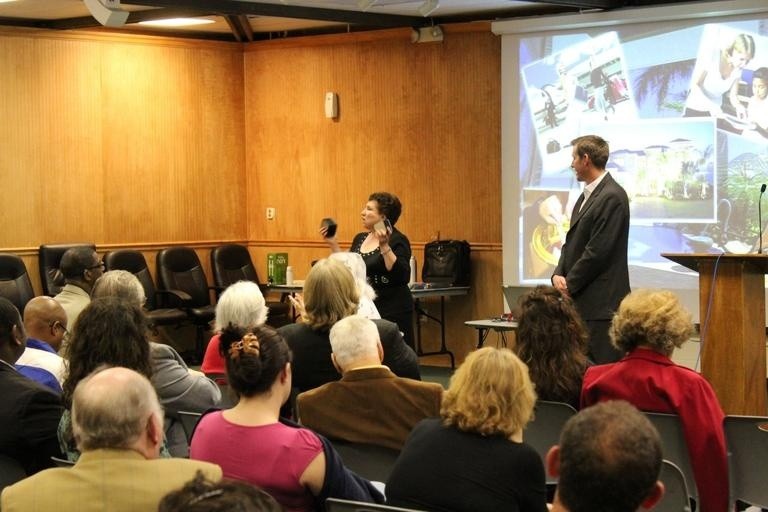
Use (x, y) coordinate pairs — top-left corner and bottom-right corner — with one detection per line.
(757, 184), (766, 254)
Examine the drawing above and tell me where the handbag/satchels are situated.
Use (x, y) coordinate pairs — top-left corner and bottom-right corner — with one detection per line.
(422, 239), (471, 289)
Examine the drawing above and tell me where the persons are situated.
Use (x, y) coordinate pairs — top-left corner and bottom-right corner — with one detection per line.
(158, 476), (282, 512)
(385, 346), (549, 512)
(551, 134), (630, 362)
(1, 366), (223, 512)
(202, 259), (422, 395)
(546, 399), (666, 512)
(189, 321), (385, 512)
(558, 58), (610, 131)
(512, 284), (594, 414)
(582, 288), (729, 512)
(318, 192), (415, 352)
(683, 34), (768, 143)
(2, 246), (221, 490)
(297, 314), (446, 491)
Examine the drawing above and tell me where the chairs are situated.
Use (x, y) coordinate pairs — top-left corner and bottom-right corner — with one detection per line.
(507, 396), (578, 482)
(37, 247), (109, 333)
(718, 412), (767, 512)
(628, 411), (699, 509)
(1, 253), (51, 365)
(158, 245), (221, 326)
(104, 249), (180, 336)
(209, 244), (296, 330)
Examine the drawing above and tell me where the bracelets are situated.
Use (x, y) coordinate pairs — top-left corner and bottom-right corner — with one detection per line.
(382, 247), (392, 255)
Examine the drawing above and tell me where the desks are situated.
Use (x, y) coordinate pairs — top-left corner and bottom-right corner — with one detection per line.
(269, 271), (459, 375)
(464, 318), (521, 355)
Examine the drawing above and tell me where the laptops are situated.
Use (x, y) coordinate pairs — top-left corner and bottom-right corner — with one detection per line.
(502, 287), (551, 325)
